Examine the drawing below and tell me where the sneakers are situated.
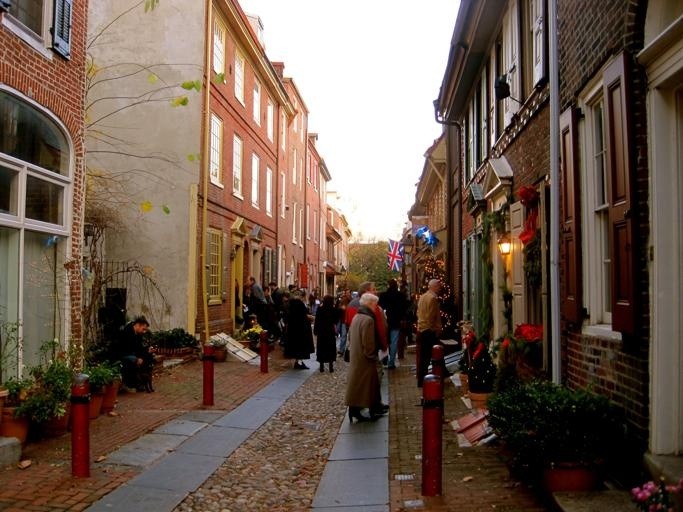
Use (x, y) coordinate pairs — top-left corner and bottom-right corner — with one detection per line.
(293, 361), (334, 373)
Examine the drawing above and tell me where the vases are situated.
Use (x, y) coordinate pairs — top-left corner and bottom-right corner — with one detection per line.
(469, 392), (488, 412)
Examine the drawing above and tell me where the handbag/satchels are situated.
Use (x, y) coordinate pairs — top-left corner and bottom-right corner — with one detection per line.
(343, 348), (349, 362)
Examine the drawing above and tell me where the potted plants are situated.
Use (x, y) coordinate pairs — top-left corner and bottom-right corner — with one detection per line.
(209, 339), (229, 361)
(0, 329), (118, 448)
(489, 382), (623, 492)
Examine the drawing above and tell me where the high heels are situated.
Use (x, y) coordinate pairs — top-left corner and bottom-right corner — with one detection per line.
(368, 404), (389, 417)
(348, 410), (369, 424)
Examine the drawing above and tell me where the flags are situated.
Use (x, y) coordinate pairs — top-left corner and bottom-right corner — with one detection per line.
(388, 239), (404, 272)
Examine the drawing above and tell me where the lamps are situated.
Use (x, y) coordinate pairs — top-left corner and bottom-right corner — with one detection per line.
(495, 230), (511, 256)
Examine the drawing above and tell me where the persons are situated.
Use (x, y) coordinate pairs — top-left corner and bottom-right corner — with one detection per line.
(242, 279), (410, 376)
(344, 294), (389, 423)
(106, 315), (157, 393)
(416, 279), (454, 388)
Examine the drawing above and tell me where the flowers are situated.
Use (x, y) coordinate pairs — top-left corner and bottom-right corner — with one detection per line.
(459, 323), (545, 391)
